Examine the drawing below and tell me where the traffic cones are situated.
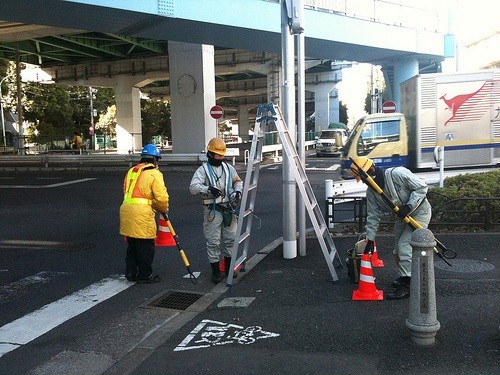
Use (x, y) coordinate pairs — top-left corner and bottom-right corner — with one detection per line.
(220, 257), (240, 272)
(155, 213), (178, 247)
(351, 253), (384, 301)
(366, 240), (385, 268)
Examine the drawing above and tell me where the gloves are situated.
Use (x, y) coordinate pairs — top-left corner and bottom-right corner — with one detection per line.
(364, 239), (374, 255)
(229, 191), (240, 204)
(395, 205), (411, 220)
(207, 186), (222, 198)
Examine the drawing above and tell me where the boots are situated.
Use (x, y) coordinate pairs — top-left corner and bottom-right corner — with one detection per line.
(391, 275), (402, 289)
(223, 256), (237, 279)
(211, 261), (222, 283)
(386, 276), (412, 300)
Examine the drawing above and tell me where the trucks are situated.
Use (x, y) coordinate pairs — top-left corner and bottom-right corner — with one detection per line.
(340, 69), (500, 179)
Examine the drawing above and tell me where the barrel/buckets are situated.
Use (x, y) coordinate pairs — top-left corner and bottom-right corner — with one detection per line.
(344, 248), (371, 283)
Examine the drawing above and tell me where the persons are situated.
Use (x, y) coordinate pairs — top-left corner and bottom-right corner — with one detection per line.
(119, 144), (169, 281)
(350, 155), (432, 300)
(189, 138), (243, 283)
(69, 132), (82, 154)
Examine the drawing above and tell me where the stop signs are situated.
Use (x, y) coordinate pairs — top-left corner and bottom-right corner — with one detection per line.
(210, 106), (223, 119)
(382, 100), (396, 113)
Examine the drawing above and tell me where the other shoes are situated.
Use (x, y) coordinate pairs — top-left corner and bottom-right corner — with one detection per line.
(131, 273), (160, 284)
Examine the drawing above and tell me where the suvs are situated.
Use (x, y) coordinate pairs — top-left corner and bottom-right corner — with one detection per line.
(315, 129), (347, 156)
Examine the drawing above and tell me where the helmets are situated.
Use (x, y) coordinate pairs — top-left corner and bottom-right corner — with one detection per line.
(349, 157), (373, 183)
(141, 144), (162, 159)
(207, 138), (226, 155)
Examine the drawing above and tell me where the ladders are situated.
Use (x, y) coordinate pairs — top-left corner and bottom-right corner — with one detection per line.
(226, 104), (343, 288)
